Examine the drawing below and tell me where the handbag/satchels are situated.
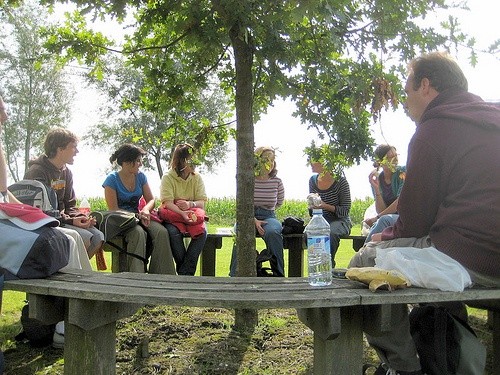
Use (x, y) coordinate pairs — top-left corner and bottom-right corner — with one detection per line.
(282, 217), (306, 234)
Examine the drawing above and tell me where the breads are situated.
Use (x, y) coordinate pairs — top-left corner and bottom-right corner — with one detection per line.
(192, 214), (197, 221)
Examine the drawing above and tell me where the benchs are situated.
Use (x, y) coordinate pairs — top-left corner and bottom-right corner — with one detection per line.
(3, 232), (500, 375)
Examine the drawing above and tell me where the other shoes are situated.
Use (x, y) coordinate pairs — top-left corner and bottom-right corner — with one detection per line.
(363, 360), (426, 375)
(52, 332), (65, 349)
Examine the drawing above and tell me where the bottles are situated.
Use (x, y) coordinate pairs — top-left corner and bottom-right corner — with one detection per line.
(306, 208), (333, 286)
(79, 195), (90, 218)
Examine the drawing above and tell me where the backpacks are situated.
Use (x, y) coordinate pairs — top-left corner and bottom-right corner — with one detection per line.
(8, 177), (58, 217)
(88, 211), (139, 247)
(410, 305), (486, 375)
(256, 248), (285, 278)
(1, 203), (70, 279)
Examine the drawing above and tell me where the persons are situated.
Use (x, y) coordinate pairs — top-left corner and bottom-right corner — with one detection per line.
(346, 50), (500, 375)
(0, 95), (8, 196)
(306, 148), (352, 268)
(364, 144), (408, 246)
(157, 144), (208, 276)
(229, 146), (286, 277)
(7, 190), (93, 335)
(102, 144), (177, 274)
(24, 128), (104, 258)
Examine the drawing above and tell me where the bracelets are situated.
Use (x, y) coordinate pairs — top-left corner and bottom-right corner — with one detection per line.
(1, 191), (7, 196)
(377, 215), (380, 219)
(193, 202), (197, 207)
